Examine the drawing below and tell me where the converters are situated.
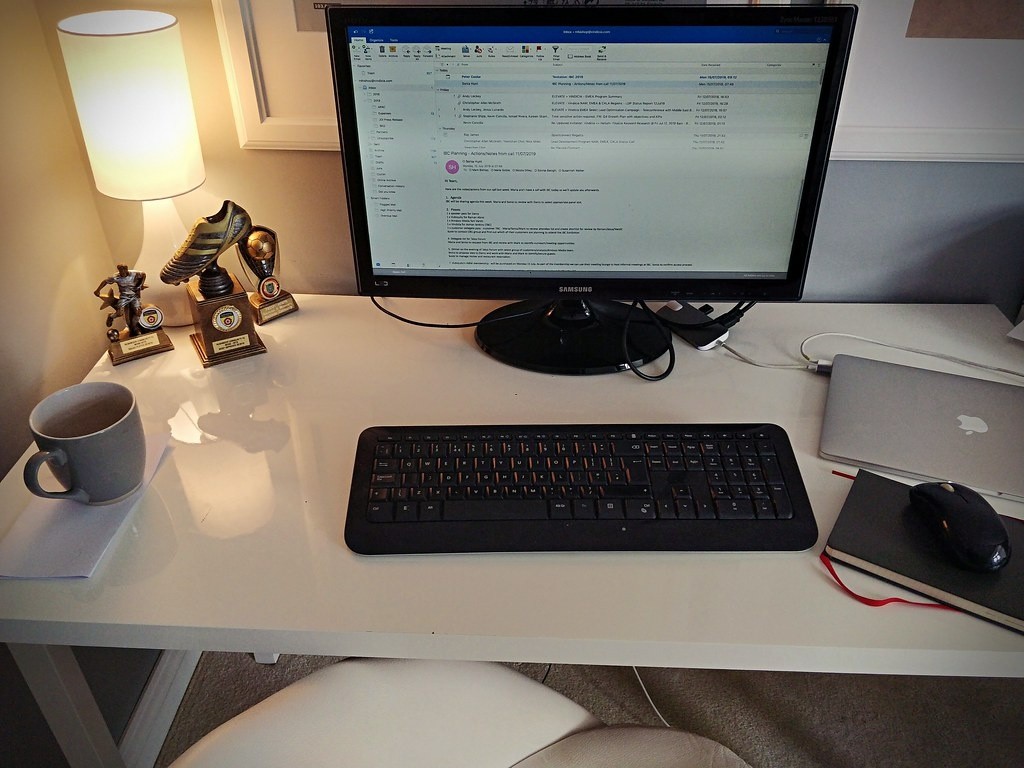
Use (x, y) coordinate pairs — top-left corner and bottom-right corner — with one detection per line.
(657, 302), (729, 351)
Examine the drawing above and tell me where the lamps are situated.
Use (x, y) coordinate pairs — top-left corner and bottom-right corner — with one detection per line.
(54, 7), (210, 328)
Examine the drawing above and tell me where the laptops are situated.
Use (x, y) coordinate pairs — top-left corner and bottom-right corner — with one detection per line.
(817, 353), (1024, 502)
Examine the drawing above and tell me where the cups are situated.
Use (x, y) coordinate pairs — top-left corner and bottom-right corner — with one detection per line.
(23, 381), (149, 508)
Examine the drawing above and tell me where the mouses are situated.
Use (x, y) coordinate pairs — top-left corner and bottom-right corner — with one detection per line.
(908, 480), (1010, 572)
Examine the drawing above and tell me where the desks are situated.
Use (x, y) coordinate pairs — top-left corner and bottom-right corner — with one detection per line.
(1, 292), (1024, 768)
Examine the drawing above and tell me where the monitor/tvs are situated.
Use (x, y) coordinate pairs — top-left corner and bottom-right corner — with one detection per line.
(322, 4), (859, 304)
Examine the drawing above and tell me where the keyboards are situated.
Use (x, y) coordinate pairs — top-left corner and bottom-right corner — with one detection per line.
(343, 423), (818, 551)
